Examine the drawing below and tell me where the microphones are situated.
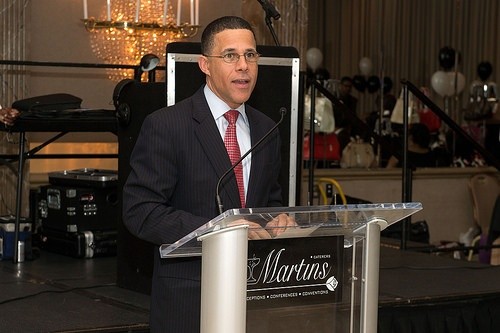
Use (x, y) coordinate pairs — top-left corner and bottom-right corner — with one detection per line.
(257, 0), (280, 20)
(215, 108), (288, 215)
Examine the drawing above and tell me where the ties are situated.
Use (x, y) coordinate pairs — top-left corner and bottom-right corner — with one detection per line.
(223, 109), (245, 208)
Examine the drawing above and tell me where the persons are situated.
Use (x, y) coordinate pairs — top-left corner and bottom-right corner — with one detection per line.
(122, 15), (299, 332)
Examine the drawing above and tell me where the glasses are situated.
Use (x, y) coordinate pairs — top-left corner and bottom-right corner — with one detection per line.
(203, 50), (262, 62)
